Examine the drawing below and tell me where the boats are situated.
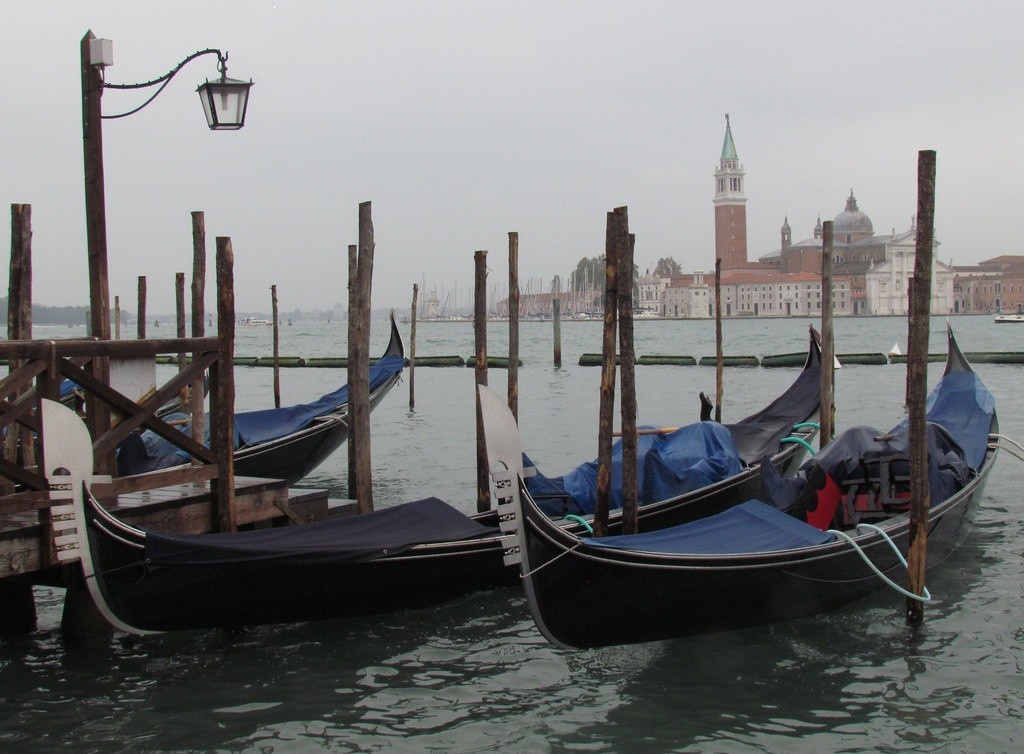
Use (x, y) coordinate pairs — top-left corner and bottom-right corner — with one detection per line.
(39, 324), (842, 637)
(241, 317), (273, 327)
(478, 315), (1002, 651)
(0, 305), (406, 486)
(994, 312), (1024, 324)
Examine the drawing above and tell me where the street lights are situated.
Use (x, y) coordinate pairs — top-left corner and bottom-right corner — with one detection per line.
(70, 26), (257, 477)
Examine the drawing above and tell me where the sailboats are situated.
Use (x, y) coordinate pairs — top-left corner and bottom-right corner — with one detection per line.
(418, 263), (607, 322)
